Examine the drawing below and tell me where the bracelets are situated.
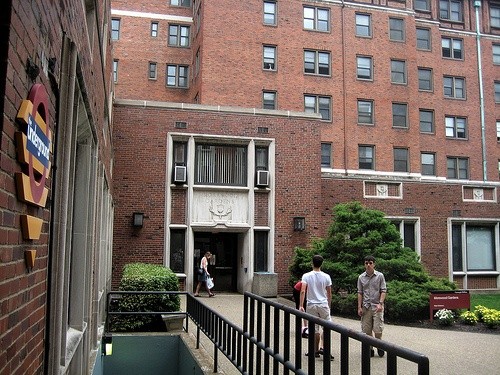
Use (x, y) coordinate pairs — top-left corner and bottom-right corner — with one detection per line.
(380, 300), (384, 305)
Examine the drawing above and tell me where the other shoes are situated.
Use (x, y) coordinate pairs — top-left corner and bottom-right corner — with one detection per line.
(317, 348), (335, 360)
(371, 349), (375, 357)
(305, 350), (320, 358)
(377, 348), (385, 357)
(194, 294), (202, 297)
(208, 293), (217, 298)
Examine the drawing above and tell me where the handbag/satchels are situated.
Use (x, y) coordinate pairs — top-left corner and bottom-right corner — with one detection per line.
(195, 268), (204, 276)
(204, 275), (214, 290)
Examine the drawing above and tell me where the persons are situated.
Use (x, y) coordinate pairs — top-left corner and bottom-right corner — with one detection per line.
(194, 248), (215, 301)
(354, 253), (388, 358)
(291, 254), (339, 361)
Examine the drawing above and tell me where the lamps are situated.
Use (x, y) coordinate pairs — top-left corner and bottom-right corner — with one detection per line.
(294, 217), (305, 232)
(132, 212), (144, 228)
(102, 333), (112, 356)
